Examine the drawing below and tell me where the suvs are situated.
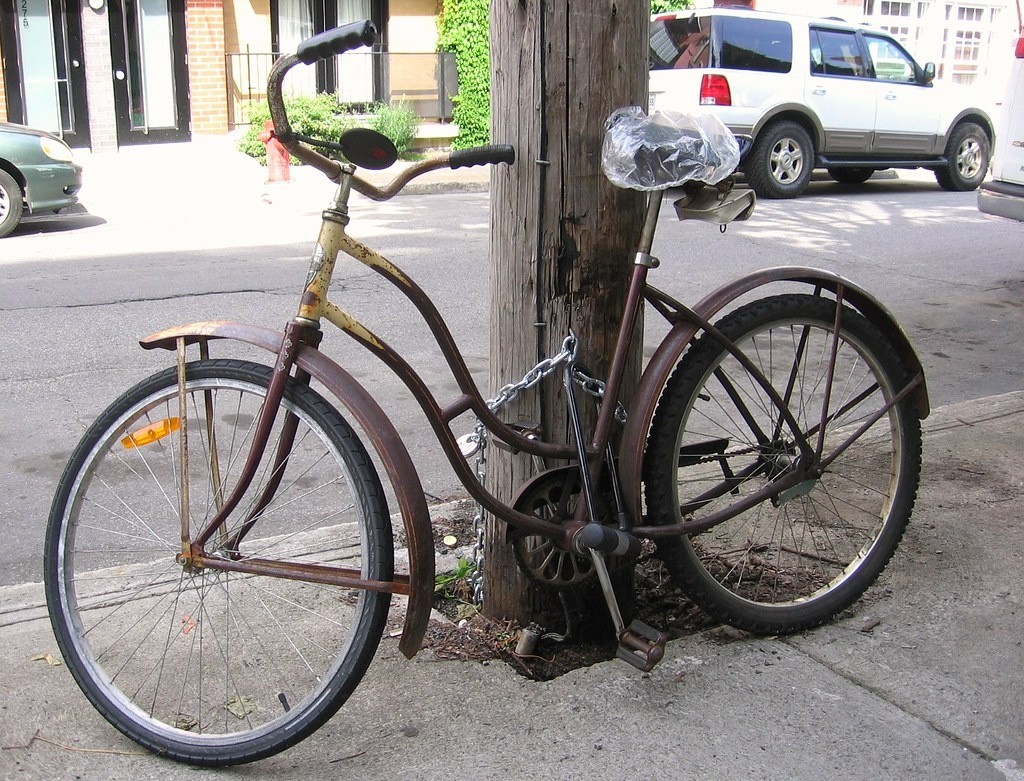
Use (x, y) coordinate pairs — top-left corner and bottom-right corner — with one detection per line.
(648, 6), (1002, 200)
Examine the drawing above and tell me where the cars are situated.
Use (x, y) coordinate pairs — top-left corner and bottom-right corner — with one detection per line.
(0, 120), (84, 239)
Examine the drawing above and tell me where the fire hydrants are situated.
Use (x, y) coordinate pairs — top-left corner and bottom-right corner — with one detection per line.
(256, 118), (292, 185)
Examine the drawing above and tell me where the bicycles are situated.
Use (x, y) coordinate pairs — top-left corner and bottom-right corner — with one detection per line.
(41, 18), (933, 769)
